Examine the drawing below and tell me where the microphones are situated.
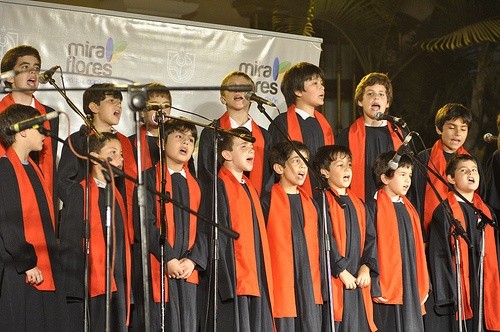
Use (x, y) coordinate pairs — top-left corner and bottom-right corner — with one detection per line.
(388, 132), (413, 170)
(38, 65), (59, 84)
(146, 103), (170, 112)
(245, 93), (276, 107)
(0, 69), (20, 81)
(375, 112), (403, 123)
(5, 110), (62, 136)
(484, 133), (500, 144)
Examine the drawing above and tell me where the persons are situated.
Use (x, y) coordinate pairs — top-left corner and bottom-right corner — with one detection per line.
(429, 153), (500, 332)
(0, 104), (71, 332)
(256, 140), (328, 332)
(363, 151), (432, 332)
(126, 83), (197, 186)
(204, 127), (277, 332)
(197, 70), (273, 229)
(57, 132), (132, 332)
(335, 72), (421, 217)
(267, 62), (336, 198)
(312, 143), (381, 332)
(412, 102), (487, 244)
(58, 83), (139, 250)
(131, 114), (210, 332)
(0, 44), (59, 242)
